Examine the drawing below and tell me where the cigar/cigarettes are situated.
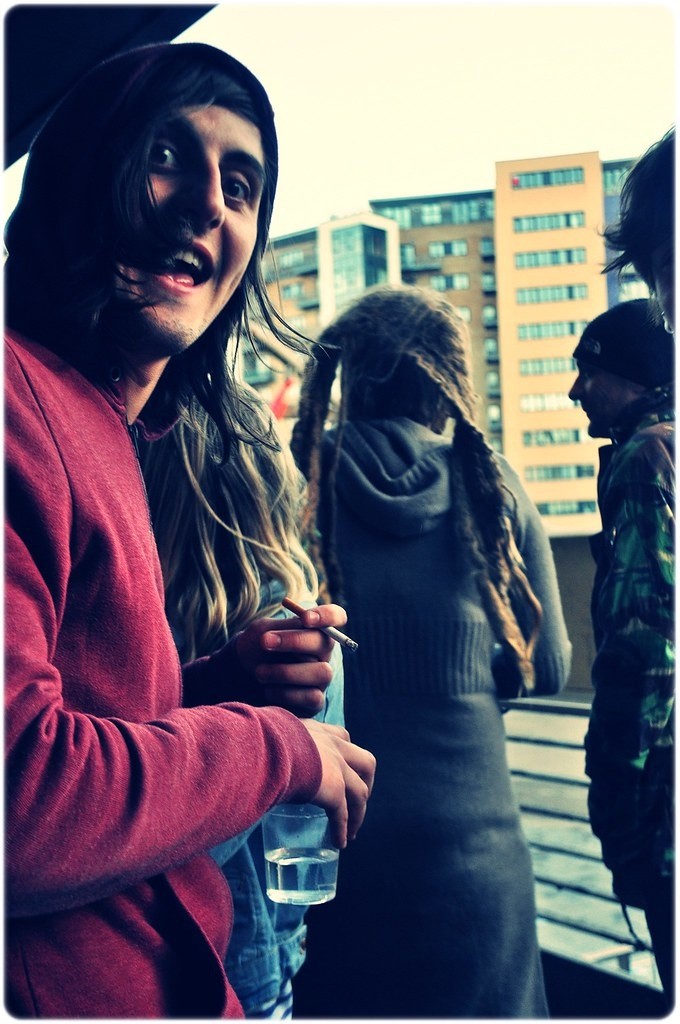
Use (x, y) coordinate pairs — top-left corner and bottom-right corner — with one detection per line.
(282, 598), (358, 654)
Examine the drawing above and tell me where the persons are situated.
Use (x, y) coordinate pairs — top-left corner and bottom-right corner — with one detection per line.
(138, 127), (675, 1018)
(5, 42), (377, 1020)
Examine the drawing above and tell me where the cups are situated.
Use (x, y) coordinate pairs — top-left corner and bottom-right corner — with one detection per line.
(260, 801), (341, 906)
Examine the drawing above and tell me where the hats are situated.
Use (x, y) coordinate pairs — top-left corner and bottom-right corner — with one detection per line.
(573, 299), (674, 387)
